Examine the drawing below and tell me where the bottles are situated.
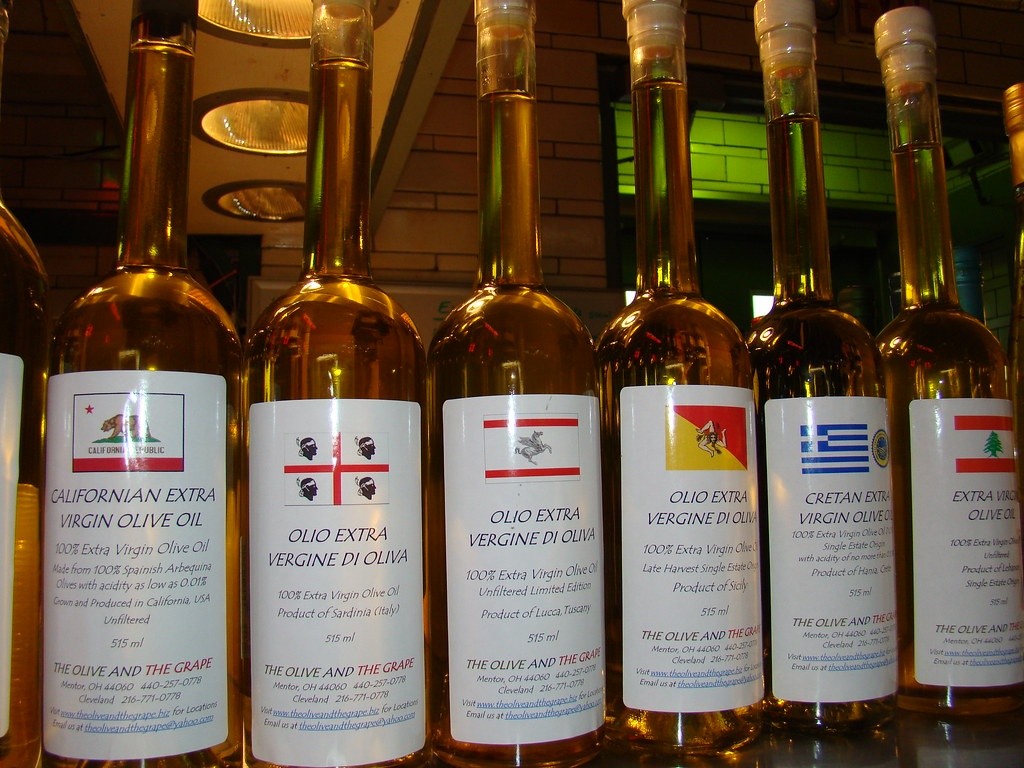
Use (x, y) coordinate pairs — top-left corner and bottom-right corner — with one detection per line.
(0, 0), (1024, 768)
